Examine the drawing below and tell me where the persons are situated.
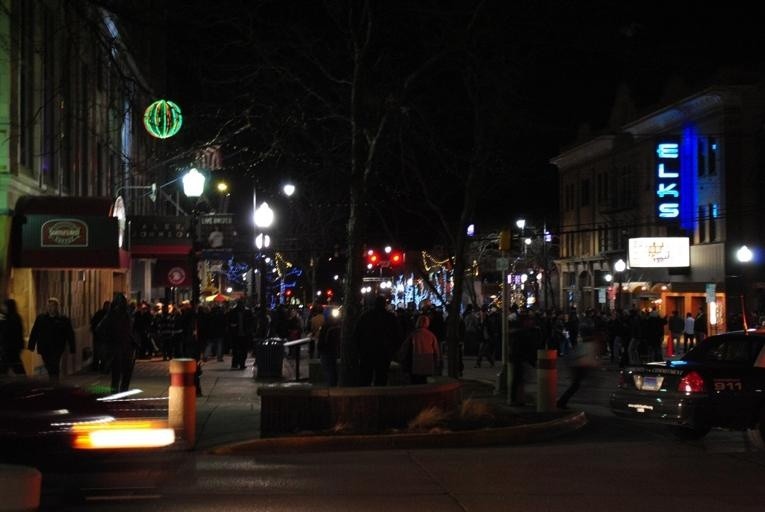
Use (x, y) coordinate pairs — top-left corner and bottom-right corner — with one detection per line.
(0, 296), (30, 380)
(88, 291), (711, 410)
(26, 295), (77, 385)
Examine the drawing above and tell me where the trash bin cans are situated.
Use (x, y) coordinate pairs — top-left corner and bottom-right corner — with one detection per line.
(256, 337), (284, 379)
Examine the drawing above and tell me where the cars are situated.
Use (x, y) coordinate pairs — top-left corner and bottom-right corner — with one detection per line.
(0, 372), (180, 511)
(612, 326), (764, 454)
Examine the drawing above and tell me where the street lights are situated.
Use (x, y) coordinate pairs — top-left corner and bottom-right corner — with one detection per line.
(736, 242), (756, 331)
(249, 198), (277, 374)
(615, 257), (625, 313)
(179, 167), (210, 399)
